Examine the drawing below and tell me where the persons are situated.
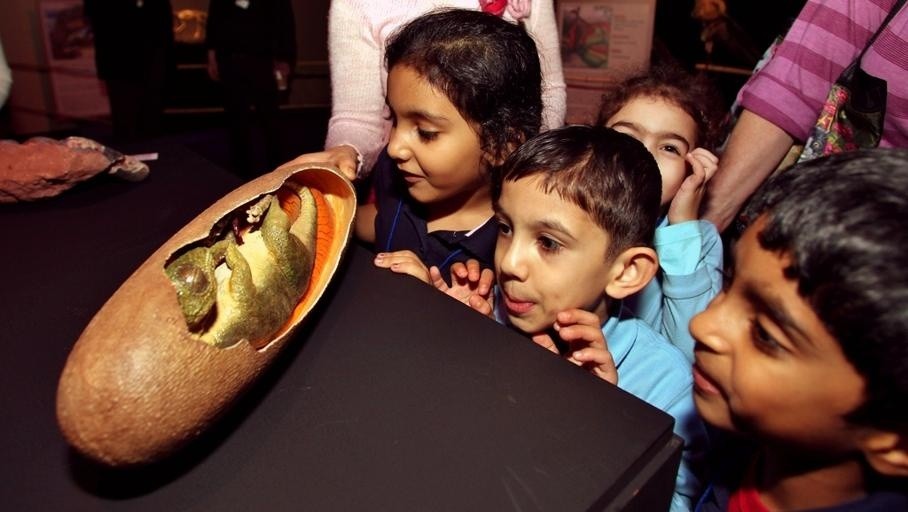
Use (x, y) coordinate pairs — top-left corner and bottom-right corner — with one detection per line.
(80, 0), (182, 147)
(430, 126), (707, 512)
(344, 5), (543, 290)
(271, 0), (568, 205)
(684, 147), (907, 511)
(592, 65), (727, 370)
(696, 0), (907, 234)
(201, 0), (298, 180)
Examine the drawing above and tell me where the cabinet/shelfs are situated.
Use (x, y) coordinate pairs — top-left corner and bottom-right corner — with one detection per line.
(0, 174), (684, 512)
